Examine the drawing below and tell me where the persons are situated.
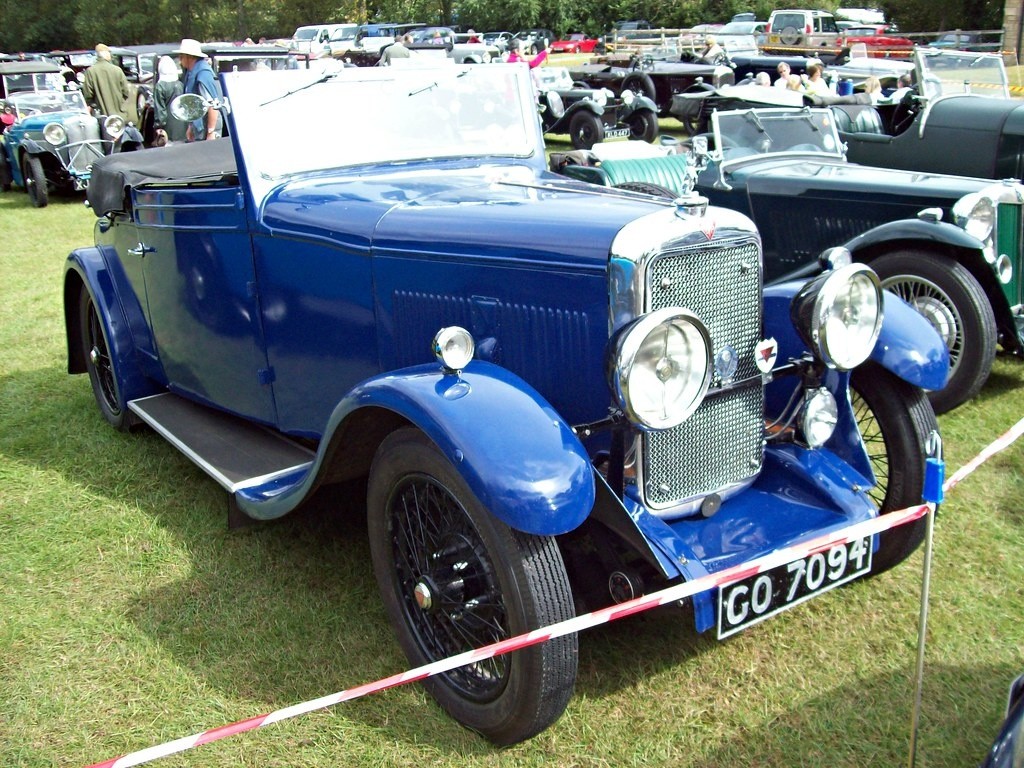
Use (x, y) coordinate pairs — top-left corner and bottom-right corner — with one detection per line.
(889, 74), (913, 104)
(380, 34), (413, 66)
(467, 29), (481, 43)
(507, 38), (552, 69)
(241, 38), (264, 46)
(154, 39), (218, 142)
(865, 77), (884, 104)
(774, 61), (832, 95)
(83, 43), (129, 124)
(433, 32), (444, 44)
(755, 72), (771, 86)
(702, 37), (716, 56)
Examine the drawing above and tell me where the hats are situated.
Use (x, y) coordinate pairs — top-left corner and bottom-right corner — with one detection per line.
(172, 39), (209, 58)
(96, 43), (111, 52)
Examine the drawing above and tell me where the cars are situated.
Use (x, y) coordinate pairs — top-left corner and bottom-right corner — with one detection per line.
(1, 7), (1023, 749)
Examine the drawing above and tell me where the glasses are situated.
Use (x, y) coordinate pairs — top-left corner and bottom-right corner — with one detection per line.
(780, 70), (786, 74)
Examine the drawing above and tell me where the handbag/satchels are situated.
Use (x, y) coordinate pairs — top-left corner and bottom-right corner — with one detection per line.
(152, 129), (169, 148)
